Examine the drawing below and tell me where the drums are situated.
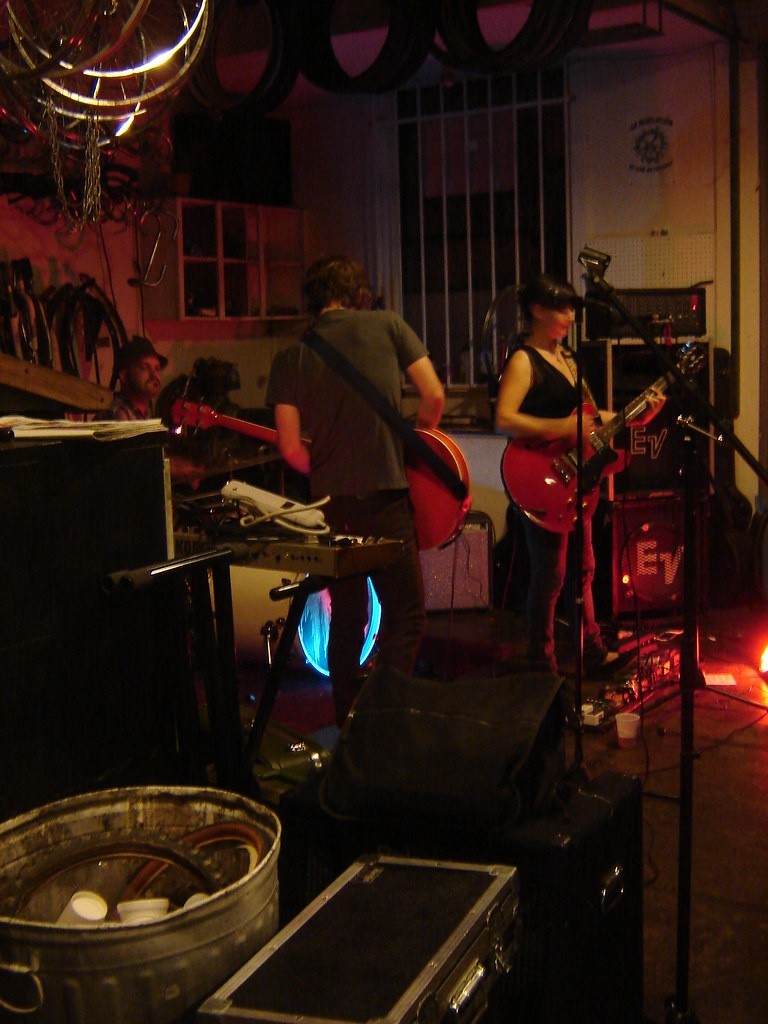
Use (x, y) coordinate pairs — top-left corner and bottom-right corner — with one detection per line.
(205, 563), (308, 675)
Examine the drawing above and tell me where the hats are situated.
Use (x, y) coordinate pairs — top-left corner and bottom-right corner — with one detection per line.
(118, 336), (168, 377)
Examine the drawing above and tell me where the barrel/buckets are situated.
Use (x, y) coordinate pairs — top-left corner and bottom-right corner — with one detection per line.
(0, 786), (283, 1024)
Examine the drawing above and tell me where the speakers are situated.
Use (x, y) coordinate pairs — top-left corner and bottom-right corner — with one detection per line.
(576, 341), (713, 622)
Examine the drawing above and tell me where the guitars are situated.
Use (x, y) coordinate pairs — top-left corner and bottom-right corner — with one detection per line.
(496, 339), (711, 539)
(169, 392), (474, 557)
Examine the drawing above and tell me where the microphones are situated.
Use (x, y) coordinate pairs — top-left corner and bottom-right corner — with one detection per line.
(546, 287), (614, 315)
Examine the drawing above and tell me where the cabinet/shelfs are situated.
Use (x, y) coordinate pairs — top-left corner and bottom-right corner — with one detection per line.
(137, 197), (314, 324)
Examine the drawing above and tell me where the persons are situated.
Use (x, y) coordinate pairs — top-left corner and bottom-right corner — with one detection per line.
(491, 273), (666, 680)
(88, 334), (171, 420)
(263, 257), (446, 732)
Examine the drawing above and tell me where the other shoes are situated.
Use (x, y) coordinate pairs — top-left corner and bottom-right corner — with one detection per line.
(589, 662), (637, 681)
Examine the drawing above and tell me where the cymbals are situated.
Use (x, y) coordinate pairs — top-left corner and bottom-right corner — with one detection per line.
(200, 501), (230, 509)
(171, 446), (287, 486)
(179, 490), (221, 502)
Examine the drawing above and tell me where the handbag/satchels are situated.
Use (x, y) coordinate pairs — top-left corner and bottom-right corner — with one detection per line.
(315, 664), (575, 841)
